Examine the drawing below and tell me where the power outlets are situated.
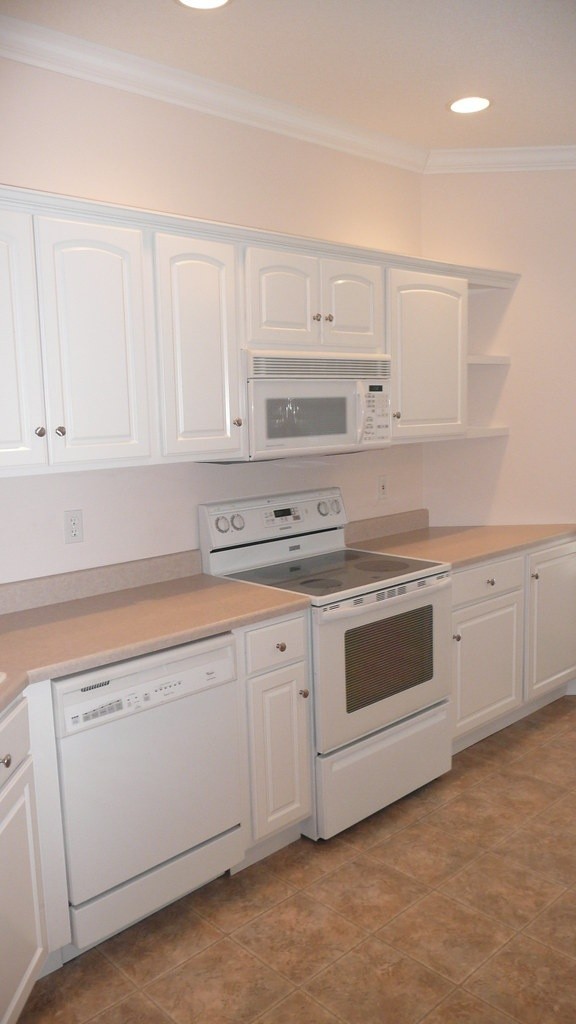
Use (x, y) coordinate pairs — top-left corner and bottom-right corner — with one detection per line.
(377, 480), (387, 500)
(63, 509), (83, 543)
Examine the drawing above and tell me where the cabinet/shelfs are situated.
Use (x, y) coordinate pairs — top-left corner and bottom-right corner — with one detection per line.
(0, 208), (150, 469)
(232, 609), (312, 847)
(385, 268), (513, 445)
(0, 693), (48, 1023)
(152, 231), (249, 465)
(451, 534), (576, 738)
(243, 246), (385, 353)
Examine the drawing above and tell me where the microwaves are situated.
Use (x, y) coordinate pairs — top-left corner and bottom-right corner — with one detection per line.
(246, 361), (393, 462)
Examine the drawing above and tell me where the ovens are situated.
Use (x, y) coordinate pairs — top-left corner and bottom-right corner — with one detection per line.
(310, 569), (452, 841)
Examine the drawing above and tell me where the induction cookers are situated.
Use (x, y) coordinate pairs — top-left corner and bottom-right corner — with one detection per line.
(197, 486), (451, 608)
